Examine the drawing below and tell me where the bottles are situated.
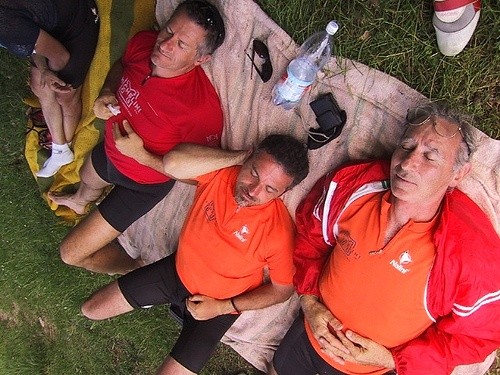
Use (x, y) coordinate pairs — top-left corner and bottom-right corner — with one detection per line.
(271, 21), (339, 111)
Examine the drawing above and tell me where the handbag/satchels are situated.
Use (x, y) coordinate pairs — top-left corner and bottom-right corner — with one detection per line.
(293, 92), (347, 150)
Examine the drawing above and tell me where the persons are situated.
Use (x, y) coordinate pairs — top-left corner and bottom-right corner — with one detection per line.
(81, 130), (311, 374)
(1, 1), (102, 179)
(264, 106), (499, 373)
(47, 0), (227, 277)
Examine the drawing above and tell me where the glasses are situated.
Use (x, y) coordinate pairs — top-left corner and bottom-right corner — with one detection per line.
(406, 108), (471, 155)
(243, 40), (273, 83)
(192, 0), (217, 27)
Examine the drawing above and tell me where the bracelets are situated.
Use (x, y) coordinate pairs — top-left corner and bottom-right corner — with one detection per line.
(231, 298), (241, 314)
